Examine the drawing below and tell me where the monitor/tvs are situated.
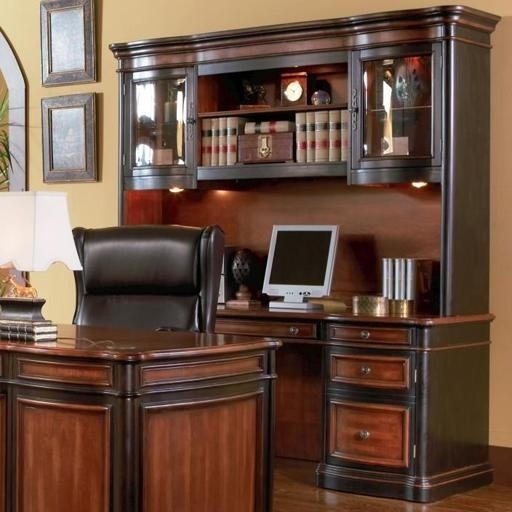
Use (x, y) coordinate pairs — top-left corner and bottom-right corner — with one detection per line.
(262, 225), (340, 309)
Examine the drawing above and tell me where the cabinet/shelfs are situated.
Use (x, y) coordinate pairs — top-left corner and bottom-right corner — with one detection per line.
(105, 5), (504, 503)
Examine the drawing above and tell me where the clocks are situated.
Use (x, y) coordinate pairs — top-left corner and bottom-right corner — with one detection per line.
(278, 72), (309, 107)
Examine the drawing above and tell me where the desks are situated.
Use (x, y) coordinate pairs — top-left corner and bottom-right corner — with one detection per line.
(1, 326), (283, 512)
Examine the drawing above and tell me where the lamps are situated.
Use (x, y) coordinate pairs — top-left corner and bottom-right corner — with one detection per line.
(1, 191), (83, 343)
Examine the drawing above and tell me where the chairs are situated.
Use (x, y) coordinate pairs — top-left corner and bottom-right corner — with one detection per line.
(72, 224), (226, 334)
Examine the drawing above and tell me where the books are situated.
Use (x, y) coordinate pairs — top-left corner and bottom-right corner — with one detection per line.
(383, 256), (432, 300)
(0, 319), (58, 343)
(201, 110), (350, 169)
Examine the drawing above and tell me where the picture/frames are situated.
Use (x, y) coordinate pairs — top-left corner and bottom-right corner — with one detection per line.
(39, 0), (98, 88)
(41, 92), (99, 184)
